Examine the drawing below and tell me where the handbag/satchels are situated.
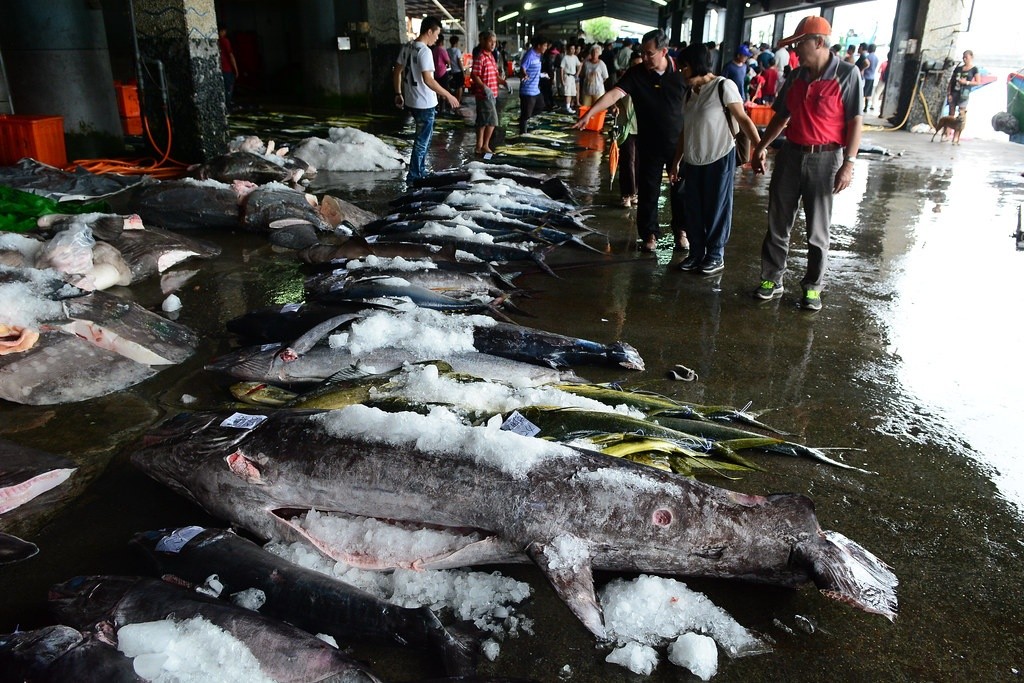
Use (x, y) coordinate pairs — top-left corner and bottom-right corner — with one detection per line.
(609, 121), (631, 145)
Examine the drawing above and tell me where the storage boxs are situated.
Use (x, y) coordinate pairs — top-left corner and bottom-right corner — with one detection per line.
(114, 79), (143, 136)
(0, 114), (66, 166)
(579, 106), (608, 131)
(746, 105), (775, 125)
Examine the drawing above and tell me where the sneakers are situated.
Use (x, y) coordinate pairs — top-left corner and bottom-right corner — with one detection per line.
(805, 290), (822, 310)
(757, 280), (784, 299)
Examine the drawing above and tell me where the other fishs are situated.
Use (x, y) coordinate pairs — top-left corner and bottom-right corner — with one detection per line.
(1, 133), (901, 683)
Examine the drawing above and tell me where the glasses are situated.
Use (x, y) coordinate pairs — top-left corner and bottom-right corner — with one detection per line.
(795, 36), (815, 47)
(639, 51), (658, 58)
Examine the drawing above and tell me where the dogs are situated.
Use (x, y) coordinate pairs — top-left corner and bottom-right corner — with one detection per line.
(931, 107), (967, 146)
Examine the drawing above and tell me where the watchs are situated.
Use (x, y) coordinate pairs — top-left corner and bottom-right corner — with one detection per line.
(844, 154), (856, 164)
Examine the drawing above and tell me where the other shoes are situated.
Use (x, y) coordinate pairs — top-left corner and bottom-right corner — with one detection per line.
(673, 230), (689, 251)
(678, 255), (707, 271)
(641, 236), (657, 253)
(566, 108), (576, 114)
(702, 257), (725, 273)
(622, 194), (638, 207)
(474, 147), (496, 155)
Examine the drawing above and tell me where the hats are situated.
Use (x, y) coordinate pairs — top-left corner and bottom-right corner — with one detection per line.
(738, 45), (754, 58)
(778, 15), (832, 45)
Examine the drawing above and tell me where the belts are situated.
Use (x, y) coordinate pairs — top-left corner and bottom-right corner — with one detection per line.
(786, 140), (841, 152)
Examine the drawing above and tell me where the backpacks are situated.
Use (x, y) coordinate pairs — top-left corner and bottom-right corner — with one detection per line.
(717, 79), (751, 166)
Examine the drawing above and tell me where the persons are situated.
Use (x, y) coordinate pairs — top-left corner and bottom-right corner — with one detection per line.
(572, 29), (692, 250)
(946, 50), (980, 139)
(218, 23), (238, 98)
(429, 29), (890, 206)
(669, 42), (767, 274)
(751, 16), (864, 309)
(395, 16), (461, 180)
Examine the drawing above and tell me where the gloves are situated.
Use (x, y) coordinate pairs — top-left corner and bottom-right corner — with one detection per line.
(504, 82), (514, 94)
(484, 86), (495, 99)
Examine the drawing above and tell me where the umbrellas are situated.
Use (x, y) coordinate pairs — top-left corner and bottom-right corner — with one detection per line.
(608, 114), (621, 190)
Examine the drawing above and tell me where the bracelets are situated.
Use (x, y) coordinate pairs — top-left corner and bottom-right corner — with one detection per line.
(967, 81), (970, 85)
(396, 93), (402, 96)
(948, 93), (952, 95)
